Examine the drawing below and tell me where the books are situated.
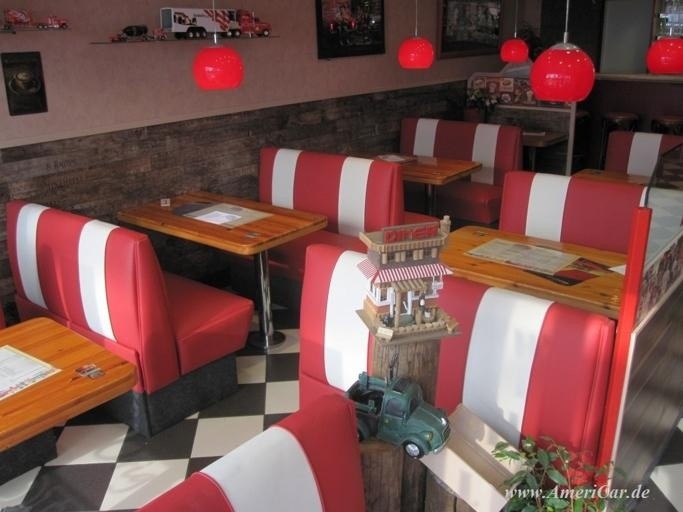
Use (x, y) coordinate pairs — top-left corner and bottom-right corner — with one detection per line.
(522, 128), (547, 136)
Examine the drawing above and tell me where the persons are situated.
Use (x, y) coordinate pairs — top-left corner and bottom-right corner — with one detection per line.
(335, 7), (350, 46)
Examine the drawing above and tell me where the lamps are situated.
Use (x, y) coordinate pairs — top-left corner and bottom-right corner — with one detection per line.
(191, 0), (243, 92)
(397, 0), (681, 104)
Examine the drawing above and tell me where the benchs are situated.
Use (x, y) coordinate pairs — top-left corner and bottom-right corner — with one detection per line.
(300, 130), (683, 506)
(4, 116), (520, 510)
